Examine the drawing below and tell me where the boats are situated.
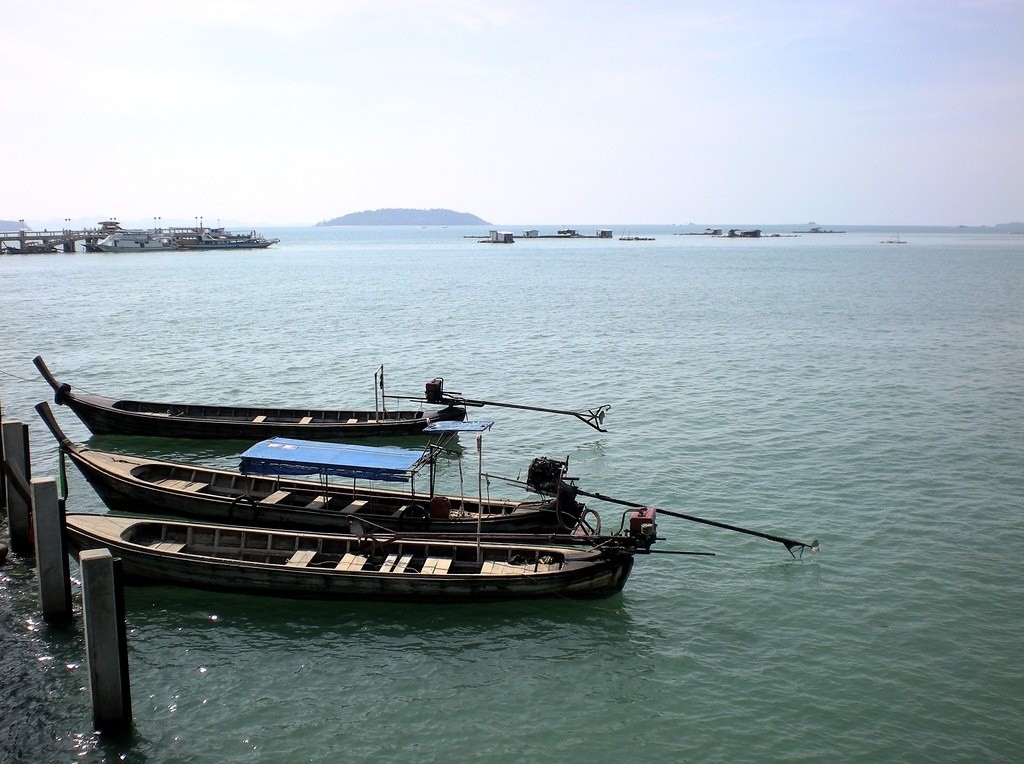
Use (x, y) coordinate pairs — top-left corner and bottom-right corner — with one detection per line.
(31, 401), (602, 538)
(81, 227), (280, 252)
(2, 241), (57, 254)
(32, 354), (485, 441)
(0, 452), (657, 607)
